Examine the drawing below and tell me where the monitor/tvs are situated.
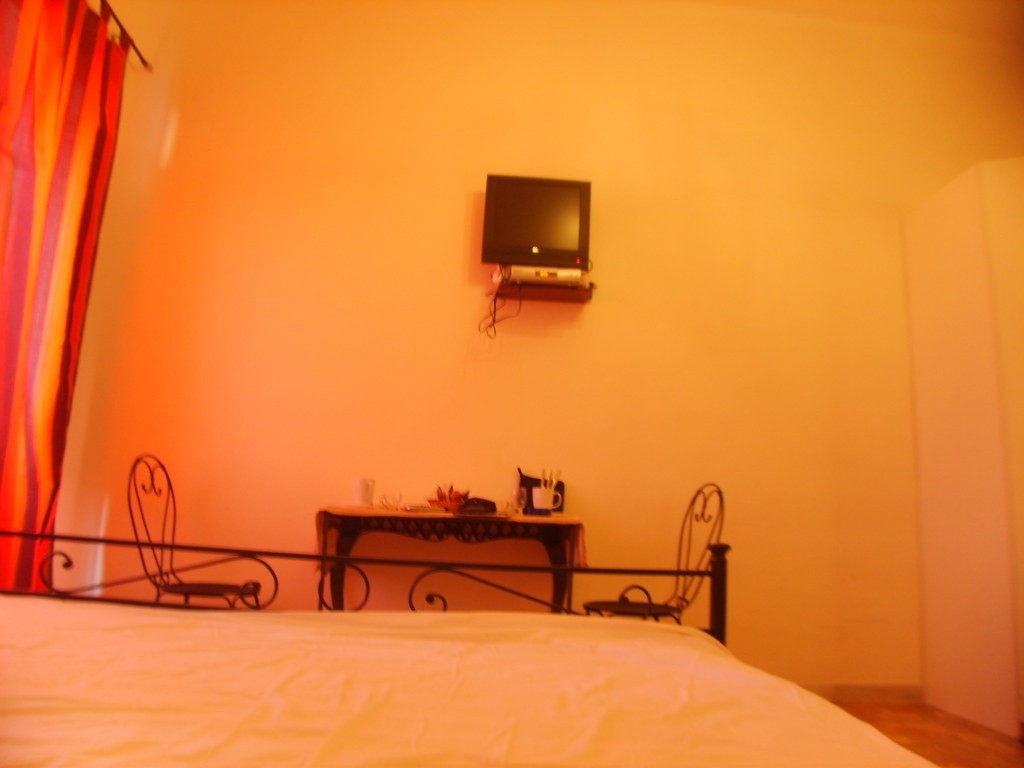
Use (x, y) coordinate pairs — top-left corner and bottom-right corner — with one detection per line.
(481, 174), (590, 270)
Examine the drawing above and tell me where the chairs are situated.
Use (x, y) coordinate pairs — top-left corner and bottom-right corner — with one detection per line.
(127, 454), (262, 609)
(582, 482), (725, 624)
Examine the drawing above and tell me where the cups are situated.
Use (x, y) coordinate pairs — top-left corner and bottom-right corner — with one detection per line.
(356, 479), (375, 507)
(533, 487), (562, 509)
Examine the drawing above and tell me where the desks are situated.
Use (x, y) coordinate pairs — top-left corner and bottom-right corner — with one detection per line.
(313, 504), (580, 613)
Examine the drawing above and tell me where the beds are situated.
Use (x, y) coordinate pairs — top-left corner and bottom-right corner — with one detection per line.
(0, 530), (937, 768)
